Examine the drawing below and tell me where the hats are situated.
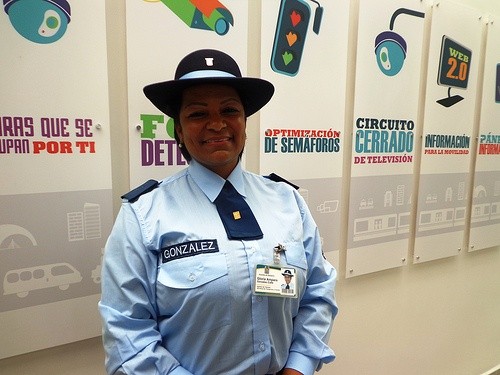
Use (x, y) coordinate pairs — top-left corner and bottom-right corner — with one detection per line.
(143, 49), (275, 120)
(282, 270), (294, 277)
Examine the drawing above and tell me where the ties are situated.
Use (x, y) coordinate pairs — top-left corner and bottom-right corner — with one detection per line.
(285, 285), (290, 289)
(215, 180), (264, 240)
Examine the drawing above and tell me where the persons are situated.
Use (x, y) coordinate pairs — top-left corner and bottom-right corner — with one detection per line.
(98, 49), (339, 375)
(280, 270), (295, 291)
